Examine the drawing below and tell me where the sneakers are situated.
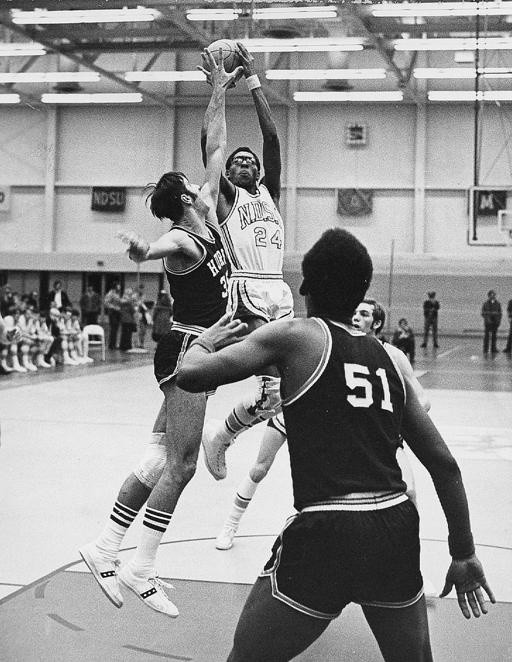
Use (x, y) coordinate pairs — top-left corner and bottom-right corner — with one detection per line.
(201, 417), (231, 480)
(216, 519), (238, 550)
(1, 357), (93, 373)
(80, 543), (123, 608)
(117, 565), (179, 618)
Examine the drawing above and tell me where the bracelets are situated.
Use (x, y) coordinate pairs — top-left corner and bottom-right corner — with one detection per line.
(245, 74), (262, 91)
(447, 532), (478, 560)
(189, 337), (216, 354)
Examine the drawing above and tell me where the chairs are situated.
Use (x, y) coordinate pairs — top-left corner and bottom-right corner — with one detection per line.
(85, 323), (106, 362)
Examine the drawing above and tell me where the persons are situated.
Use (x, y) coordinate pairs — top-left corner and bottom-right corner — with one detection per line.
(172, 223), (499, 662)
(347, 298), (435, 511)
(198, 40), (292, 549)
(0, 275), (174, 378)
(419, 290), (440, 348)
(77, 45), (245, 619)
(481, 289), (503, 355)
(502, 299), (512, 353)
(393, 317), (416, 363)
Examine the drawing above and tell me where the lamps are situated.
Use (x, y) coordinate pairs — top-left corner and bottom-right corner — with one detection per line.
(0, 0), (511, 105)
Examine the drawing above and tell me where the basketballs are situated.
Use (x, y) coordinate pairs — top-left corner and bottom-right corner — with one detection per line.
(202, 40), (243, 81)
